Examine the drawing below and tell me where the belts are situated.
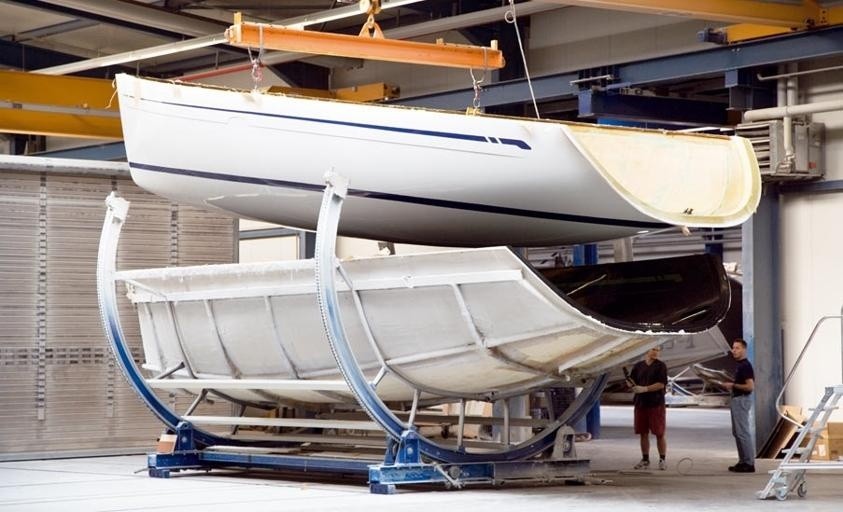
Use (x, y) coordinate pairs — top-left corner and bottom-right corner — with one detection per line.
(732, 393), (748, 398)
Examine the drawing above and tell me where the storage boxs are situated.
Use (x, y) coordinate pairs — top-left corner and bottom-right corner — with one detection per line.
(798, 421), (843, 461)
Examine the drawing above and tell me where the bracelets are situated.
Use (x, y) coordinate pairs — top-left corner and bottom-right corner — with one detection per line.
(732, 383), (735, 390)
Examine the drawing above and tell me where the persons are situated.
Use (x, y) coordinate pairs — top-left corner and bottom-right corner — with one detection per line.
(625, 345), (667, 471)
(719, 338), (755, 473)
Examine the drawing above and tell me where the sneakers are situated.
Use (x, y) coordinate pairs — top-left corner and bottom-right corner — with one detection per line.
(659, 459), (665, 468)
(728, 462), (755, 472)
(634, 459), (650, 469)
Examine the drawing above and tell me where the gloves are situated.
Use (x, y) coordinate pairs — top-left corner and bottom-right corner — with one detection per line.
(625, 377), (635, 387)
(632, 386), (648, 393)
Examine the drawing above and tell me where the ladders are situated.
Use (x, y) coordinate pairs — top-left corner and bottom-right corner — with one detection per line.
(759, 385), (843, 501)
